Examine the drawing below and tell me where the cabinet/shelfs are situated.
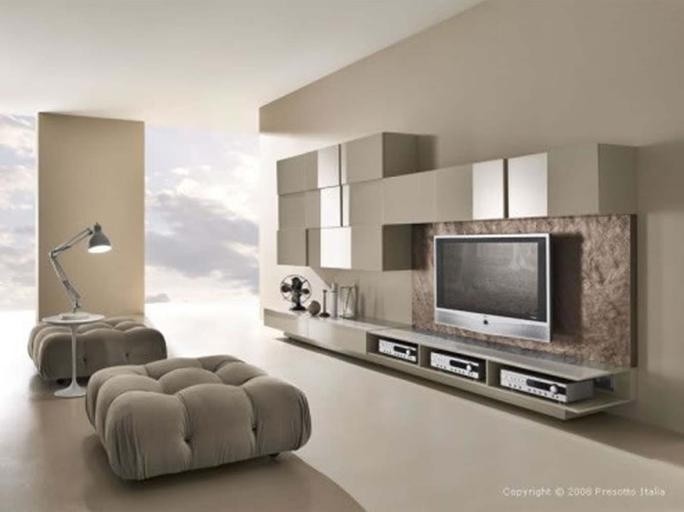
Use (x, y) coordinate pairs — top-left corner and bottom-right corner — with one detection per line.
(261, 306), (637, 423)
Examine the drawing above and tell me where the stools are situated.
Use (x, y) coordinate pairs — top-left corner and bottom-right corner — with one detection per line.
(85, 355), (310, 486)
(41, 314), (105, 398)
(27, 316), (167, 382)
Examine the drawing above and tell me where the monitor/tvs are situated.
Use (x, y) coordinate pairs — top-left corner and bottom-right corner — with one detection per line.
(431, 233), (554, 341)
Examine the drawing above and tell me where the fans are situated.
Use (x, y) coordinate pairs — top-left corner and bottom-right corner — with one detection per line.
(279, 273), (312, 311)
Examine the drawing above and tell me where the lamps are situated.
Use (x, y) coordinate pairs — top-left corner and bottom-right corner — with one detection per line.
(47, 221), (113, 320)
